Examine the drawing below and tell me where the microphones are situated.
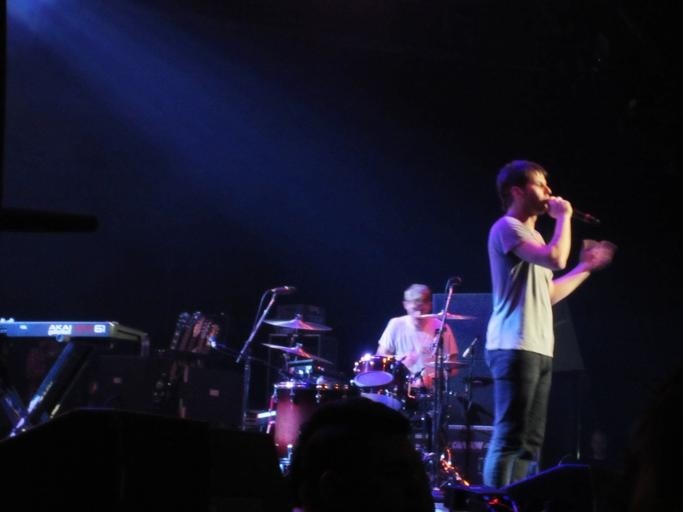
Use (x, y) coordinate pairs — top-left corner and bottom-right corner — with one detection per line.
(573, 207), (596, 224)
(272, 285), (295, 294)
(462, 338), (479, 360)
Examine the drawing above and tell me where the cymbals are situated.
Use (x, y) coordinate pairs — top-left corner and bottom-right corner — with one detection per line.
(264, 343), (332, 366)
(424, 363), (465, 368)
(263, 320), (332, 331)
(414, 312), (476, 321)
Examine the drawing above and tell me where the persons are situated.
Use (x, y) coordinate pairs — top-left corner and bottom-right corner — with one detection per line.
(375, 283), (460, 453)
(482, 160), (618, 490)
(289, 395), (435, 512)
(628, 362), (683, 512)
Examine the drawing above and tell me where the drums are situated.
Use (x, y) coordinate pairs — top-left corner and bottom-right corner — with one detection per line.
(356, 369), (396, 400)
(355, 354), (410, 397)
(276, 379), (353, 469)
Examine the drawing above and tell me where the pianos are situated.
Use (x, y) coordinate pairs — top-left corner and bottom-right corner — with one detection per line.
(1, 317), (147, 341)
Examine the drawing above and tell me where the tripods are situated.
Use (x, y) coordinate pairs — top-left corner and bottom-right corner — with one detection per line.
(424, 282), (470, 490)
